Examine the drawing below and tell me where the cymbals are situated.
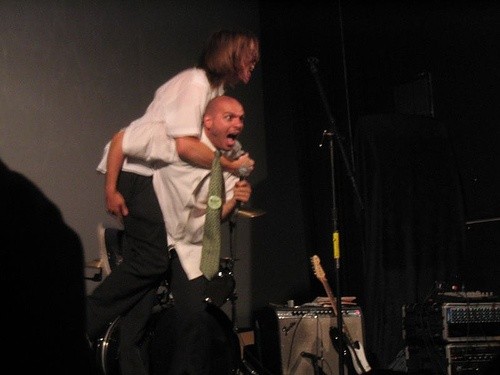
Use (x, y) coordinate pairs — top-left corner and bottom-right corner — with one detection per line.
(233, 207), (267, 219)
(84, 258), (104, 268)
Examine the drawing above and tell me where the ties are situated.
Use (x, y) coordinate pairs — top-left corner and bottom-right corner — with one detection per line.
(199, 150), (223, 280)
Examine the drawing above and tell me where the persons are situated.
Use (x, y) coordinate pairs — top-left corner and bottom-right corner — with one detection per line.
(105, 96), (252, 375)
(87, 28), (261, 342)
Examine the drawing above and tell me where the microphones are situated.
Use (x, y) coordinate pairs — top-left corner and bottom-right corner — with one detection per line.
(238, 165), (251, 206)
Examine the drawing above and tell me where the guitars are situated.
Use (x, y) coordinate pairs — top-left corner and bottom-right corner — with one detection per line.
(310, 254), (372, 375)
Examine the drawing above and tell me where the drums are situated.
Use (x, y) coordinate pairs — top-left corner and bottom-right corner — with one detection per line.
(205, 256), (237, 307)
(93, 301), (241, 375)
